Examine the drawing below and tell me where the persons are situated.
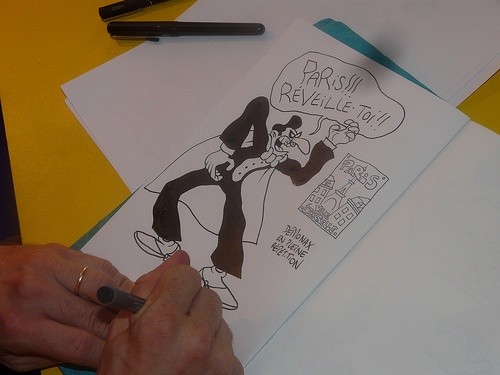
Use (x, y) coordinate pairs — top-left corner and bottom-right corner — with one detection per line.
(0, 241), (245, 375)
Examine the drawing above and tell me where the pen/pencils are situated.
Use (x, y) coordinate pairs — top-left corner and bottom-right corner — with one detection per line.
(98, 0), (169, 20)
(107, 20), (265, 42)
(97, 286), (147, 313)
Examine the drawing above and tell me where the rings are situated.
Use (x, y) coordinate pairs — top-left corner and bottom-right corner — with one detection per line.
(74, 264), (90, 296)
